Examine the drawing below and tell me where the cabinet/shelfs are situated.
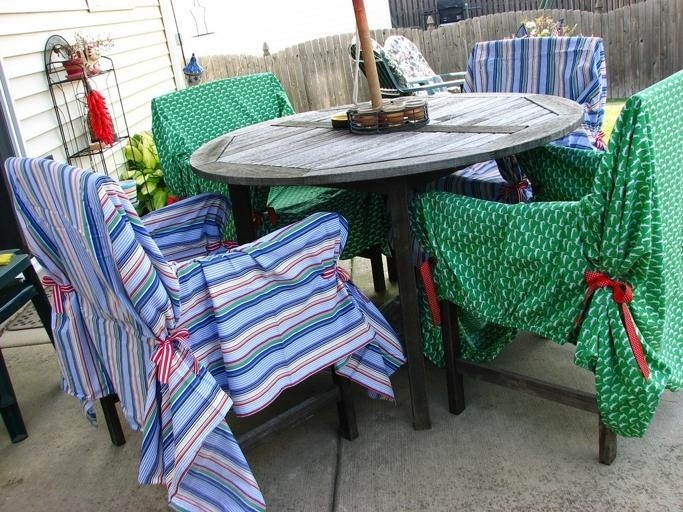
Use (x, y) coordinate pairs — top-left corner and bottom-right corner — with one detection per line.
(44, 34), (136, 177)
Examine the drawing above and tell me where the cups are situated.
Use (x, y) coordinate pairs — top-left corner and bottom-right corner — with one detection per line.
(332, 114), (350, 129)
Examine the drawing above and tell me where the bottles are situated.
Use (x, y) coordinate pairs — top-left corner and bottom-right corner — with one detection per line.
(353, 98), (427, 128)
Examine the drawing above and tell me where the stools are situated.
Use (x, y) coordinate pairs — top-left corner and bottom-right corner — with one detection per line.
(0, 249), (63, 444)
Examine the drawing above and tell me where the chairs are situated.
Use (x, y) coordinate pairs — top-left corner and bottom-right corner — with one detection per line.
(348, 34), (466, 99)
(4, 156), (407, 512)
(150, 72), (393, 294)
(408, 70), (683, 466)
(444, 36), (607, 204)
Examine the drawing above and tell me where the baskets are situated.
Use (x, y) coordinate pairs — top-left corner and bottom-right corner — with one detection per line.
(346, 103), (429, 136)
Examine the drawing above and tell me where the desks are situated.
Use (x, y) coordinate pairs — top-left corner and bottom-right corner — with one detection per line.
(189, 92), (586, 431)
